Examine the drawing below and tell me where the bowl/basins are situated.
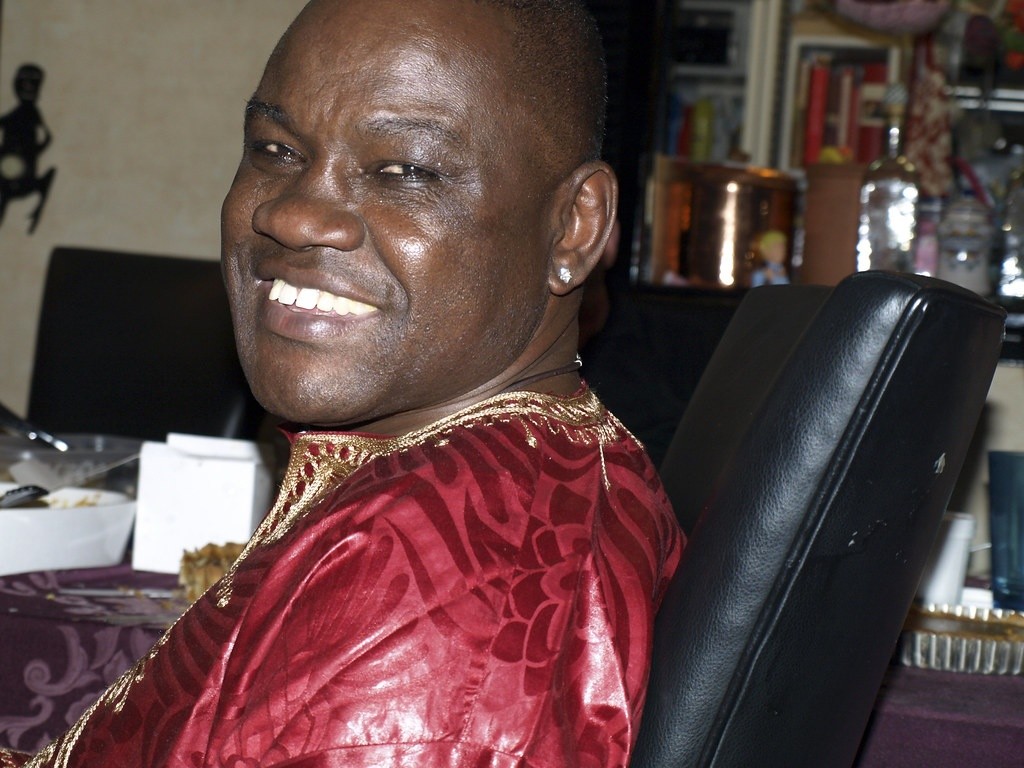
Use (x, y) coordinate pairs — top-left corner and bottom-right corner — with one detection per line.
(0, 435), (147, 494)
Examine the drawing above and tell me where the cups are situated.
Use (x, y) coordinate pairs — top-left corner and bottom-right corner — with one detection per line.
(989, 450), (1024, 608)
(917, 513), (976, 610)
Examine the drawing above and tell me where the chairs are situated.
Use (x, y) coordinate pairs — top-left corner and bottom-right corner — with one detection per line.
(624, 270), (1006, 768)
(25, 248), (266, 441)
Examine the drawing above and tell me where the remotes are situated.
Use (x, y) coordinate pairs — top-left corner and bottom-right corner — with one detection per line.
(0, 486), (49, 508)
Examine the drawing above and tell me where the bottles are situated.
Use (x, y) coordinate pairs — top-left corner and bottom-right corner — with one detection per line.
(856, 85), (922, 277)
(937, 190), (993, 295)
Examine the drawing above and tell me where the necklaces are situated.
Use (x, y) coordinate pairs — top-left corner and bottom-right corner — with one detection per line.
(505, 354), (583, 391)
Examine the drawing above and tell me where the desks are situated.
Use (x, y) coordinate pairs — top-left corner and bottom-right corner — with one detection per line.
(1, 544), (190, 759)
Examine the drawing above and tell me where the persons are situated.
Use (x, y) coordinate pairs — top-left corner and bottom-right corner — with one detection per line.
(0, 0), (690, 768)
